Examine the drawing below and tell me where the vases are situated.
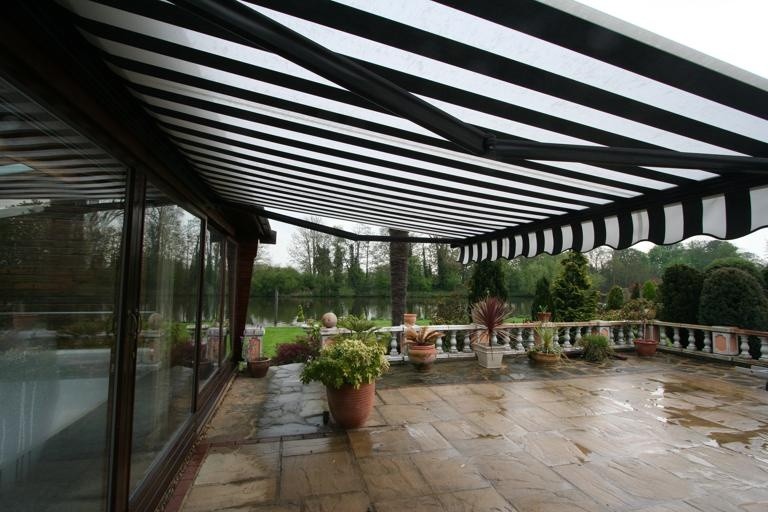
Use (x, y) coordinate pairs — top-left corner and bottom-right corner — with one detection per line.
(243, 355), (271, 378)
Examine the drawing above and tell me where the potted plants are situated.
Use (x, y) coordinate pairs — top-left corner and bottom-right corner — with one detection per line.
(464, 298), (517, 369)
(298, 339), (390, 429)
(402, 325), (446, 374)
(528, 323), (562, 362)
(537, 304), (552, 323)
(622, 298), (665, 356)
